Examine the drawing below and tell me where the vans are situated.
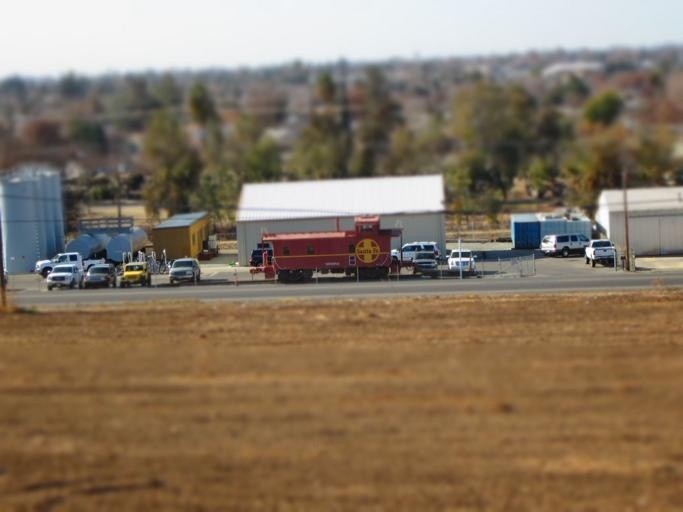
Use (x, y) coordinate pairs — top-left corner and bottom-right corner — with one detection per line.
(539, 233), (590, 258)
(247, 246), (274, 271)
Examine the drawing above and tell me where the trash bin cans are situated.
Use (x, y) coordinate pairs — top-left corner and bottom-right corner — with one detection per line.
(391, 256), (400, 275)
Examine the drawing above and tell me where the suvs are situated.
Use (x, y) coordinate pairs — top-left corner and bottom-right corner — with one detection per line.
(165, 256), (202, 284)
(81, 262), (118, 289)
(44, 264), (84, 290)
(118, 260), (152, 288)
(391, 241), (441, 264)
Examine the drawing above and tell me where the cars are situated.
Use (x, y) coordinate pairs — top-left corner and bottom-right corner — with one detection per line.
(407, 250), (441, 275)
(445, 248), (479, 273)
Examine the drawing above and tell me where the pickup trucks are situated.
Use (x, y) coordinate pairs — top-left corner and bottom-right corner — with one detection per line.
(584, 238), (618, 268)
(35, 251), (106, 278)
